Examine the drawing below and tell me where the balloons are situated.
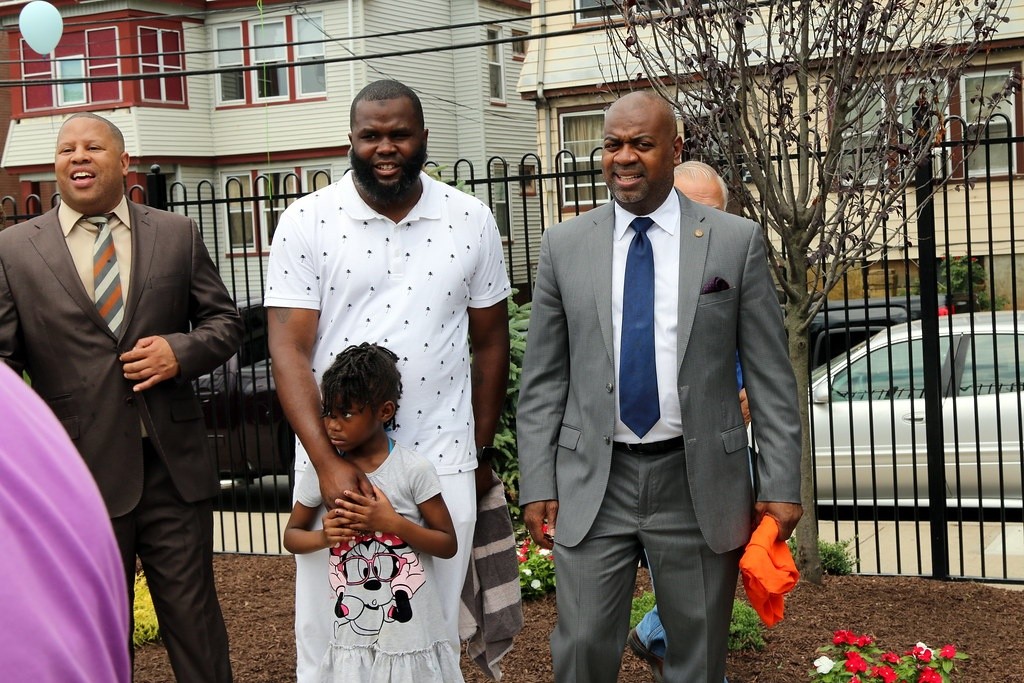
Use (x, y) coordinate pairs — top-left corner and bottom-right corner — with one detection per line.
(18, 1), (64, 57)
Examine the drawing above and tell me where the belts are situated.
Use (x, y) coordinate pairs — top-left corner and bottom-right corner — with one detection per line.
(613, 436), (684, 457)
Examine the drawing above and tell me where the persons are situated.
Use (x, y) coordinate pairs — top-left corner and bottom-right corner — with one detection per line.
(263, 80), (511, 683)
(515, 91), (804, 683)
(1, 360), (130, 683)
(0, 113), (244, 683)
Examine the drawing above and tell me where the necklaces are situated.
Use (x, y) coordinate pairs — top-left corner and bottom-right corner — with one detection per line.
(387, 436), (393, 454)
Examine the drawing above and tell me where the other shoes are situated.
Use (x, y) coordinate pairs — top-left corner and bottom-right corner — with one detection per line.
(628, 628), (665, 683)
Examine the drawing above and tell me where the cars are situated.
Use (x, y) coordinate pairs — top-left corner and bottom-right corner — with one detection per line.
(190, 295), (294, 508)
(743, 308), (1024, 519)
(777, 290), (953, 370)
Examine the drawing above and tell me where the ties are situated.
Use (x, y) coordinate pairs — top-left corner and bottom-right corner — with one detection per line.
(618, 217), (661, 439)
(86, 212), (125, 337)
